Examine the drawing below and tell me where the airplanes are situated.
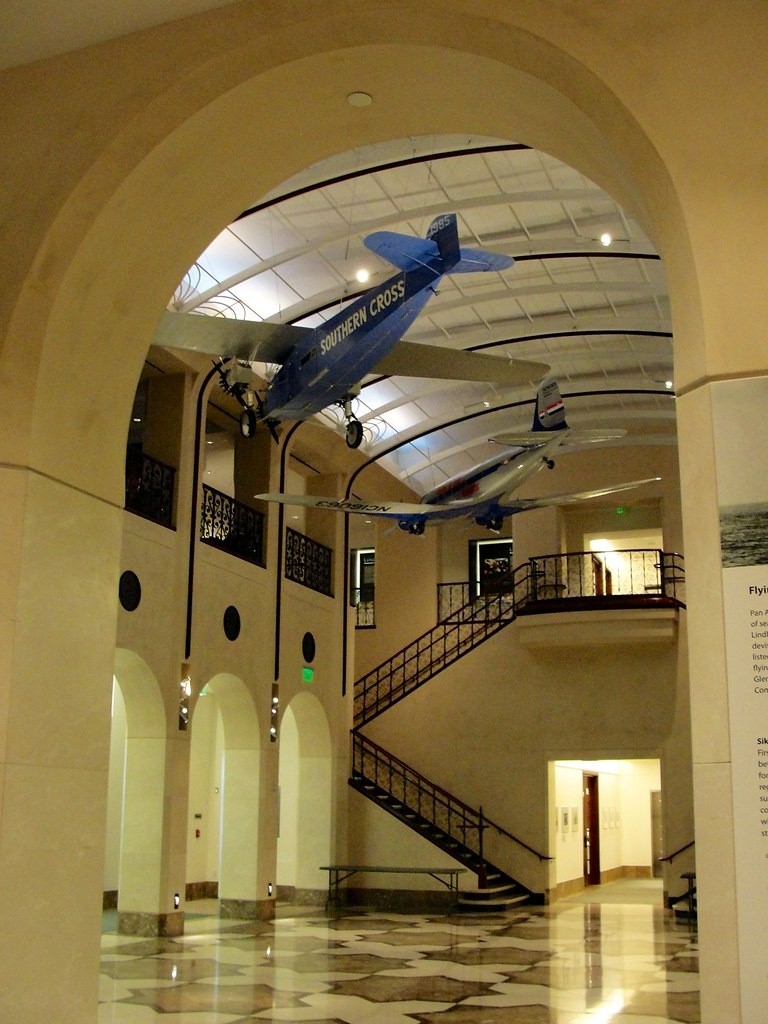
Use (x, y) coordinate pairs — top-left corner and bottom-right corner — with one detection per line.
(256, 375), (663, 537)
(148, 213), (550, 449)
